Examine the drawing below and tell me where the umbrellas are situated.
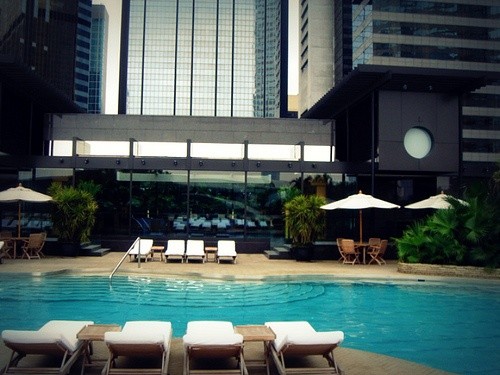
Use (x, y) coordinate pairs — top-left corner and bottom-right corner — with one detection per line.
(405, 191), (470, 209)
(0, 183), (54, 238)
(319, 190), (401, 244)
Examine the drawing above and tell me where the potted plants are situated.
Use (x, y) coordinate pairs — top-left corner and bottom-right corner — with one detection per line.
(281, 196), (330, 262)
(47, 180), (102, 255)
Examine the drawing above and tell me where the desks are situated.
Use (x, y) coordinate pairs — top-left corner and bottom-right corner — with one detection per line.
(339, 242), (378, 264)
(234, 324), (276, 375)
(76, 324), (122, 375)
(151, 246), (165, 262)
(204, 246), (218, 262)
(0, 237), (59, 259)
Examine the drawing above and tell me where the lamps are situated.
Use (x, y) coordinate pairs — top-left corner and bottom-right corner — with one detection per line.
(59, 157), (318, 169)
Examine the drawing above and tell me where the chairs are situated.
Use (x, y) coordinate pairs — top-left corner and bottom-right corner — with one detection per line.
(336, 237), (390, 266)
(0, 231), (14, 260)
(264, 322), (344, 375)
(104, 320), (171, 375)
(20, 231), (47, 260)
(165, 240), (185, 262)
(128, 238), (155, 263)
(2, 320), (94, 375)
(182, 321), (245, 375)
(217, 240), (238, 264)
(185, 240), (206, 264)
(169, 216), (269, 233)
